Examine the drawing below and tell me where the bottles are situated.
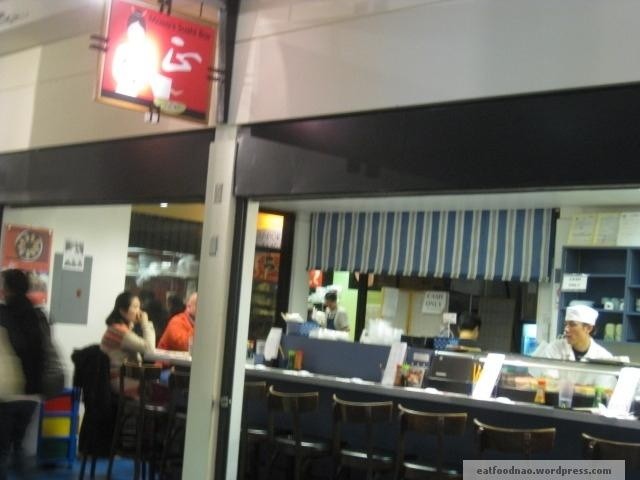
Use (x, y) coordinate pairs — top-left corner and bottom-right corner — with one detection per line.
(247, 340), (254, 360)
(534, 380), (547, 404)
(590, 386), (603, 407)
(284, 350), (304, 371)
(399, 362), (412, 387)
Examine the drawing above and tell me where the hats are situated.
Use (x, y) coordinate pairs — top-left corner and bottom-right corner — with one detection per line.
(565, 304), (600, 326)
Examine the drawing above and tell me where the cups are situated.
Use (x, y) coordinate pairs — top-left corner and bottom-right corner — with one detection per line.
(635, 297), (640, 313)
(603, 323), (615, 342)
(614, 324), (623, 341)
(599, 297), (624, 310)
(558, 378), (574, 410)
(128, 250), (181, 273)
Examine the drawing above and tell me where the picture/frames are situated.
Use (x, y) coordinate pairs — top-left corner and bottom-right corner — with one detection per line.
(565, 211), (640, 246)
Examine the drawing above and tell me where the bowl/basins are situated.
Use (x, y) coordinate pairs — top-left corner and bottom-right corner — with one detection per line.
(13, 229), (44, 262)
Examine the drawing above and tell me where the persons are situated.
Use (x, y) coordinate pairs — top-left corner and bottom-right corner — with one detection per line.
(452, 313), (483, 353)
(153, 291), (197, 386)
(112, 11), (161, 98)
(0, 269), (66, 480)
(100, 292), (155, 400)
(324, 293), (350, 332)
(527, 304), (618, 391)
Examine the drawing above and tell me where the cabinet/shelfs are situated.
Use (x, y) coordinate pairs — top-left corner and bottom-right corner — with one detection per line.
(556, 245), (640, 345)
